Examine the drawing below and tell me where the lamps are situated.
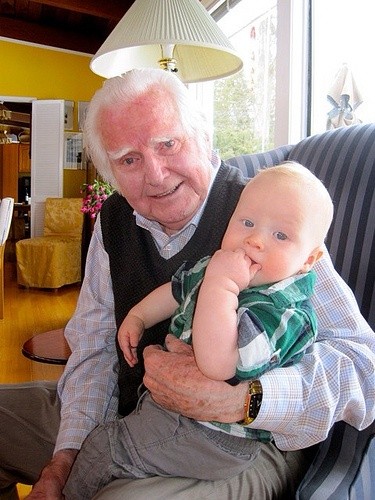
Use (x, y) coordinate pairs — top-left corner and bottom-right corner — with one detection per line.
(89, 0), (244, 85)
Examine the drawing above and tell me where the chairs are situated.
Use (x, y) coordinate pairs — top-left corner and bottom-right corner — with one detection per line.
(14, 198), (84, 293)
(0, 197), (14, 319)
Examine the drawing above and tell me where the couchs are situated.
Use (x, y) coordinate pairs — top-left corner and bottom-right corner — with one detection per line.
(218, 122), (375, 500)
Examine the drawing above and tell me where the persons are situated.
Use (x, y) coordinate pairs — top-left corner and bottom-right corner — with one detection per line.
(1, 161), (335, 499)
(58, 67), (375, 500)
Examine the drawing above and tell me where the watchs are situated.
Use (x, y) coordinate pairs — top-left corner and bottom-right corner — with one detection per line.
(235, 380), (263, 426)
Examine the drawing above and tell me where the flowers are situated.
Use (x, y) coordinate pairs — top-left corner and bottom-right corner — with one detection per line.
(80, 170), (118, 220)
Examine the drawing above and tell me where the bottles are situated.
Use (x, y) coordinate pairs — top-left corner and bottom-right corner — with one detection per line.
(24, 188), (28, 205)
